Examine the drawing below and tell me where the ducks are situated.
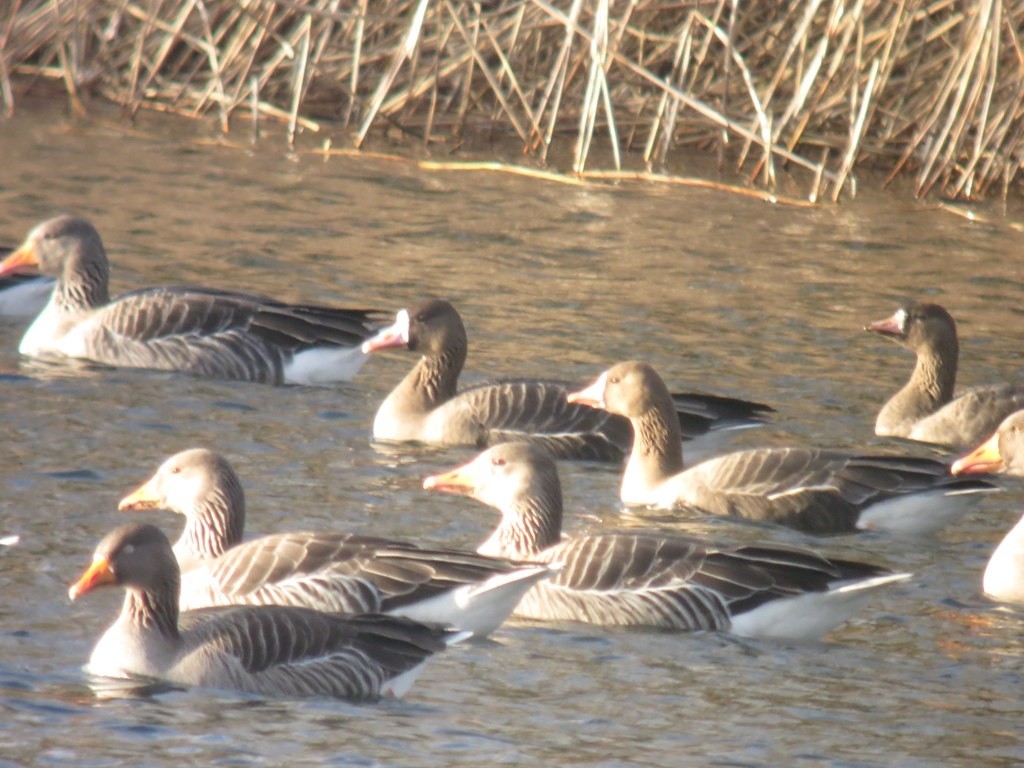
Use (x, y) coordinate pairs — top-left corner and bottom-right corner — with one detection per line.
(360, 298), (778, 464)
(421, 438), (919, 642)
(565, 359), (1008, 535)
(65, 522), (473, 701)
(948, 411), (1024, 603)
(864, 304), (1024, 440)
(0, 213), (374, 381)
(115, 447), (564, 638)
